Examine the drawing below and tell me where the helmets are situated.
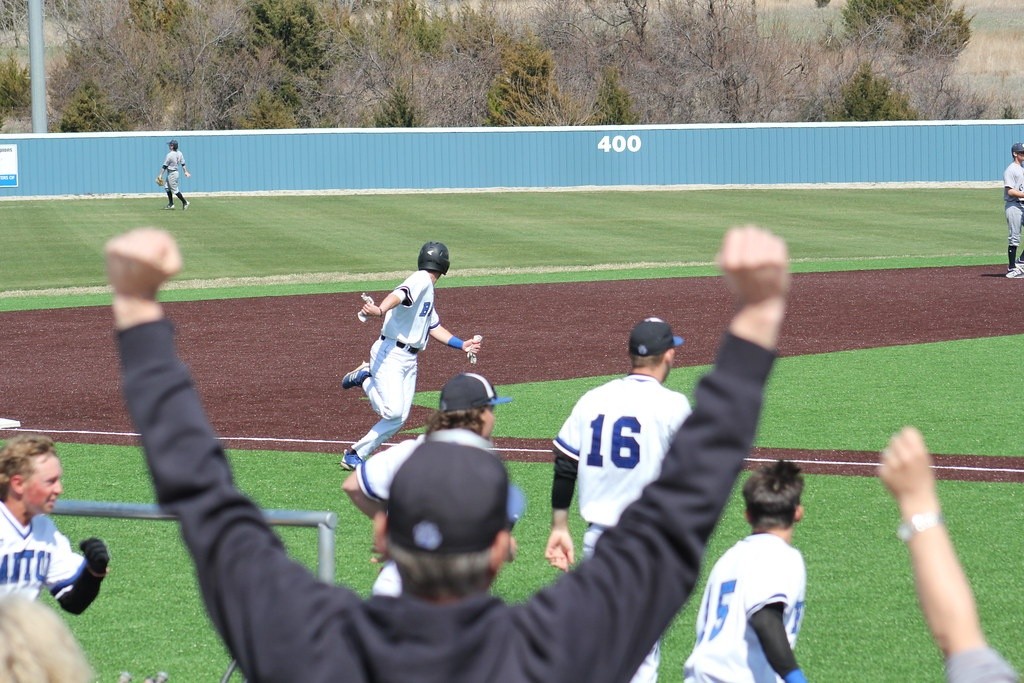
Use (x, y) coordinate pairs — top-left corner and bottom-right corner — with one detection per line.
(166, 139), (178, 150)
(418, 242), (450, 273)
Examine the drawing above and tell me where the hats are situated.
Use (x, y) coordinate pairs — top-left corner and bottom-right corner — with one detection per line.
(628, 318), (685, 355)
(438, 371), (512, 410)
(1012, 142), (1024, 152)
(387, 427), (523, 552)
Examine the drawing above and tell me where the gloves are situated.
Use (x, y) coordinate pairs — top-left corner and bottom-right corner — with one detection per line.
(463, 335), (483, 365)
(80, 538), (110, 573)
(357, 292), (374, 323)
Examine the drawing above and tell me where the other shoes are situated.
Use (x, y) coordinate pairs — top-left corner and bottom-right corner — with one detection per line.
(1006, 268), (1024, 278)
(1015, 258), (1024, 273)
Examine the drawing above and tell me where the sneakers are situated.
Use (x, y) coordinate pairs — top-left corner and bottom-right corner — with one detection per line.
(164, 204), (174, 210)
(341, 449), (363, 469)
(182, 200), (190, 211)
(341, 362), (371, 389)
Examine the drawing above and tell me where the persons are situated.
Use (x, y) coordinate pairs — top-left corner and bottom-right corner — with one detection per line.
(878, 425), (1024, 683)
(340, 240), (483, 470)
(0, 434), (109, 683)
(543, 315), (693, 683)
(340, 371), (520, 597)
(157, 139), (191, 211)
(681, 457), (812, 683)
(1004, 142), (1024, 274)
(103, 225), (790, 683)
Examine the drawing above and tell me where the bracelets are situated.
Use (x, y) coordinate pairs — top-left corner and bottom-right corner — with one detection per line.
(896, 512), (942, 540)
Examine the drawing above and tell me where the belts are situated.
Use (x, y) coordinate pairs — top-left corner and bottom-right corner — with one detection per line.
(1011, 201), (1024, 204)
(378, 335), (421, 355)
